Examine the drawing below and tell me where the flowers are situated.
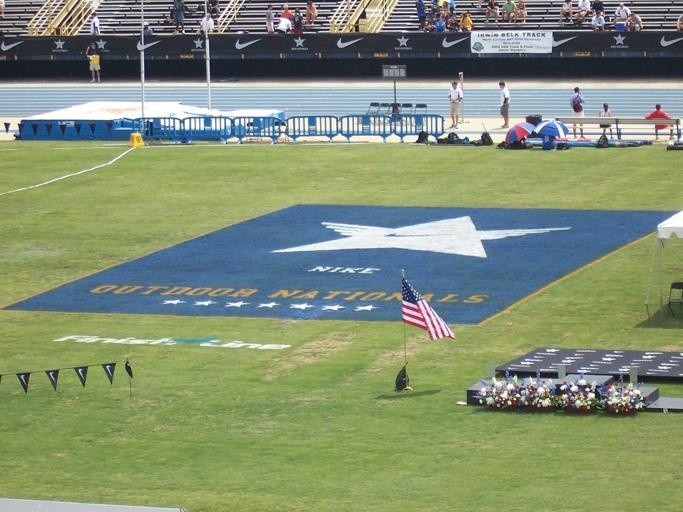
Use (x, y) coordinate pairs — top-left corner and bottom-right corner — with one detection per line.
(477, 373), (644, 415)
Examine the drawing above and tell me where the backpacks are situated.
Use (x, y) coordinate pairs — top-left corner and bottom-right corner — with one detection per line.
(596, 133), (609, 148)
(572, 94), (583, 113)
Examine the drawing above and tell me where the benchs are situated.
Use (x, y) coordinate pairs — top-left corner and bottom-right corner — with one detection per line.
(0, 0), (683, 39)
(552, 116), (681, 144)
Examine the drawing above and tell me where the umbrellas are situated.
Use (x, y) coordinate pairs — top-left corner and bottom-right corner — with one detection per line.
(533, 120), (569, 139)
(505, 123), (535, 144)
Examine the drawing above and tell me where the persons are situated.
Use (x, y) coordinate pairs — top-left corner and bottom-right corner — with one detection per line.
(87, 42), (102, 83)
(476, 0), (527, 23)
(570, 87), (585, 139)
(266, 0), (318, 34)
(90, 13), (100, 35)
(416, 0), (473, 33)
(448, 82), (463, 129)
(144, 0), (249, 35)
(499, 81), (510, 129)
(644, 104), (675, 140)
(599, 103), (613, 139)
(677, 14), (683, 32)
(558, 0), (643, 32)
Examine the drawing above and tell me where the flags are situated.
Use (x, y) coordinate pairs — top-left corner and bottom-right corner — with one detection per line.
(402, 275), (455, 341)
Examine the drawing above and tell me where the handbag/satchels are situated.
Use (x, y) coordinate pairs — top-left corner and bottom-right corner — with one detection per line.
(543, 140), (569, 150)
(497, 137), (532, 149)
(415, 130), (429, 144)
(438, 132), (493, 146)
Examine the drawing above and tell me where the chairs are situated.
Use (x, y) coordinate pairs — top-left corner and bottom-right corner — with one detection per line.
(364, 102), (429, 117)
(667, 281), (683, 323)
